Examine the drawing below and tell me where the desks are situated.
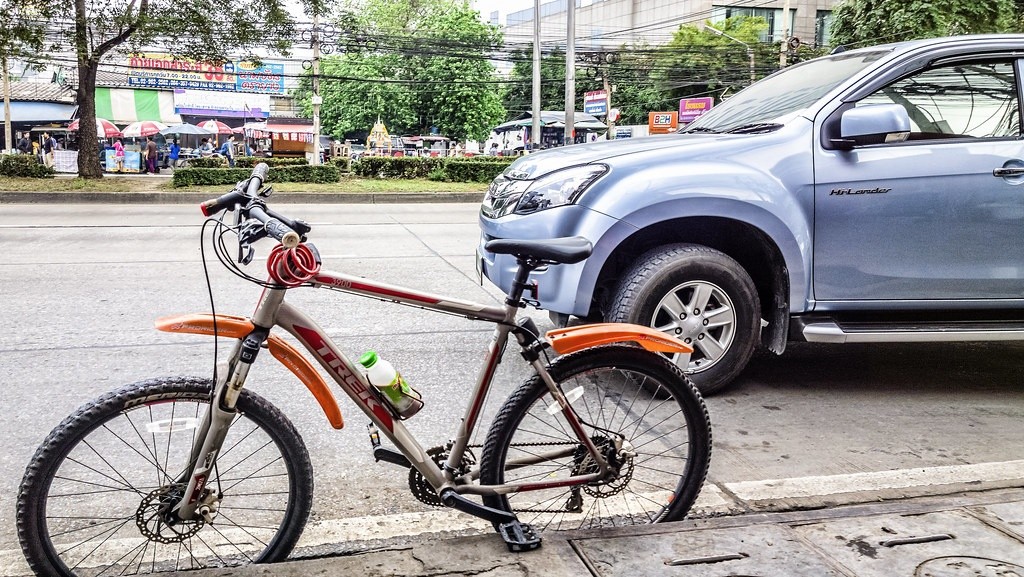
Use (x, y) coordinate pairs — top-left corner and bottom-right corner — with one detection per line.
(179, 154), (197, 167)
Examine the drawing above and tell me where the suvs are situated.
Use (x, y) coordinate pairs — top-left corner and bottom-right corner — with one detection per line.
(476, 32), (1024, 399)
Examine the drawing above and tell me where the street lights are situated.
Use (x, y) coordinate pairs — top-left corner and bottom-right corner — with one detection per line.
(705, 25), (753, 88)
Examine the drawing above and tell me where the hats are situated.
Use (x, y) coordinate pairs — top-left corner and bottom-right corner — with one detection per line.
(58, 138), (63, 142)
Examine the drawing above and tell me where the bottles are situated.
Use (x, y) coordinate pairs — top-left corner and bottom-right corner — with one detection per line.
(360, 352), (420, 418)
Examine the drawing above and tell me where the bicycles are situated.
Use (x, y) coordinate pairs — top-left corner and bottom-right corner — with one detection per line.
(15, 161), (714, 577)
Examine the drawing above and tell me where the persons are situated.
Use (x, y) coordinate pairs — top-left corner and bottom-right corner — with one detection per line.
(450, 141), (465, 157)
(247, 144), (256, 158)
(489, 142), (499, 157)
(194, 137), (217, 158)
(144, 137), (180, 175)
(113, 138), (125, 174)
(17, 131), (56, 171)
(225, 134), (235, 168)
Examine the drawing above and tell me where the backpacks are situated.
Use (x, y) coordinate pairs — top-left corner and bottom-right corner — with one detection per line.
(221, 143), (228, 155)
(51, 137), (56, 150)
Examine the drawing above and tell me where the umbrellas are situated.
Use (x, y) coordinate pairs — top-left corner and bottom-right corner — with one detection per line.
(68, 117), (234, 152)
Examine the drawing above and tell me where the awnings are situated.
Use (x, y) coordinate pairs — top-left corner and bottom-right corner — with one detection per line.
(244, 123), (324, 135)
(494, 119), (546, 134)
(410, 135), (451, 142)
(0, 101), (79, 121)
(94, 88), (183, 126)
